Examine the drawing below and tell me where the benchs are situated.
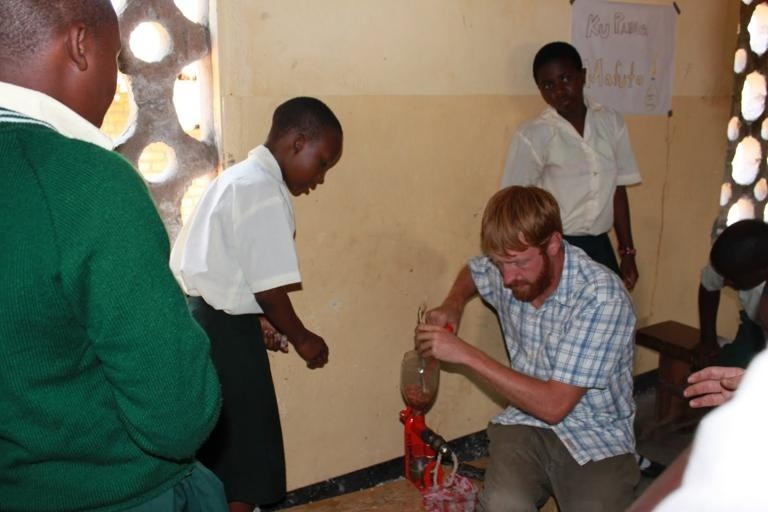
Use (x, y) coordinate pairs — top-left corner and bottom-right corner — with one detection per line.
(635, 320), (734, 421)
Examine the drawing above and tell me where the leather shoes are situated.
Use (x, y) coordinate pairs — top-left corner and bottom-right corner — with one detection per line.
(638, 455), (666, 477)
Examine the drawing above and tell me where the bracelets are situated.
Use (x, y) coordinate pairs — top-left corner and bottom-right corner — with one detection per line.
(618, 247), (636, 257)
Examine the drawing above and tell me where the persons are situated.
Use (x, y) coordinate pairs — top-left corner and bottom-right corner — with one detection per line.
(167, 96), (344, 512)
(0, 0), (227, 512)
(414, 187), (642, 512)
(500, 42), (664, 478)
(689, 219), (767, 368)
(630, 347), (766, 512)
